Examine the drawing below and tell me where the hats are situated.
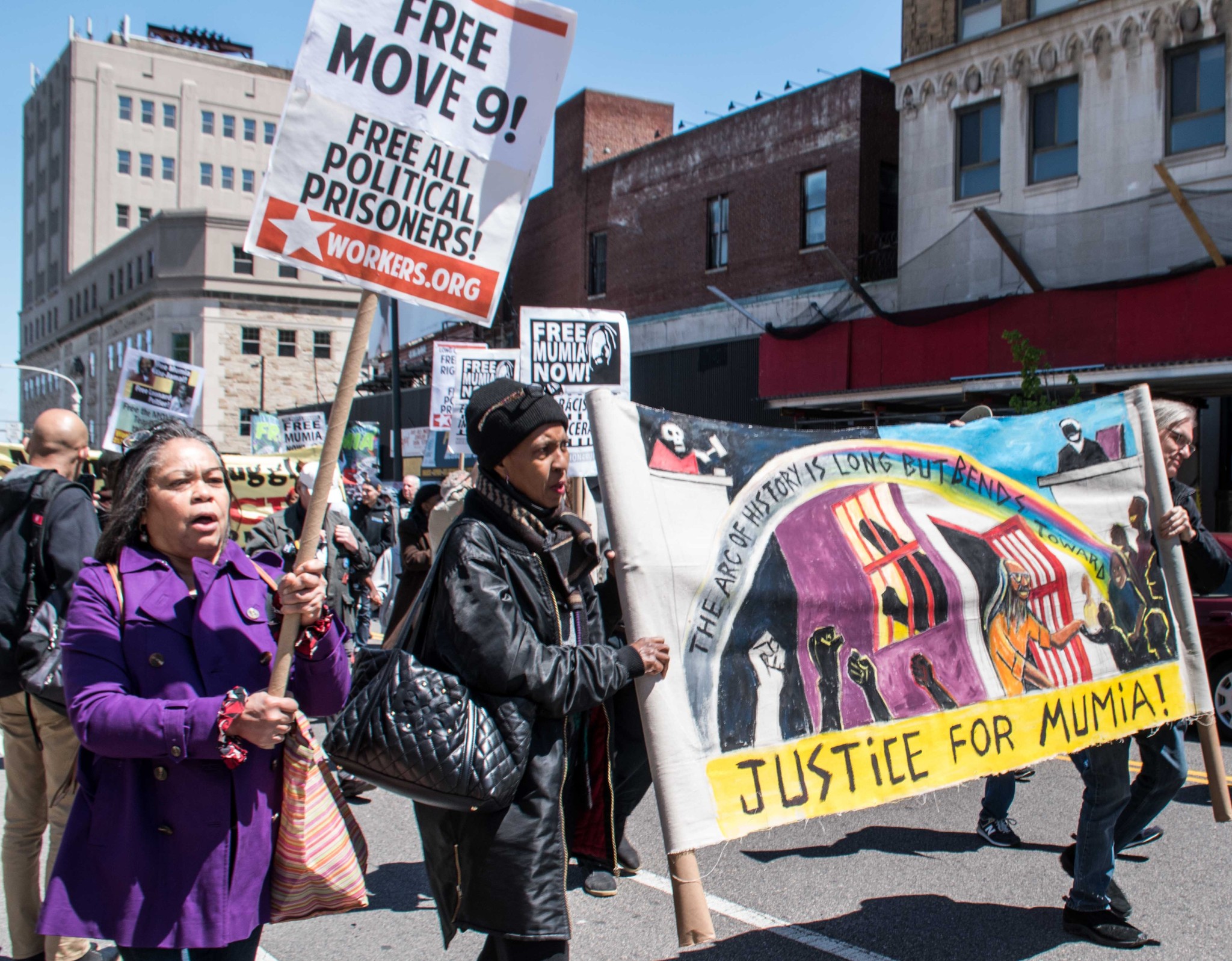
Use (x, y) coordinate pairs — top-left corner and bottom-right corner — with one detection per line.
(465, 376), (568, 468)
(363, 476), (382, 491)
(299, 462), (344, 504)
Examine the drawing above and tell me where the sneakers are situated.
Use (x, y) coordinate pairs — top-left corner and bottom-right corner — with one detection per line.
(976, 814), (1022, 848)
(1062, 905), (1148, 949)
(1060, 843), (1133, 917)
(1124, 824), (1164, 848)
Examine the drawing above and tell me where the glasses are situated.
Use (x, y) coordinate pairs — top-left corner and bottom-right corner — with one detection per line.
(1164, 425), (1196, 457)
(478, 381), (562, 433)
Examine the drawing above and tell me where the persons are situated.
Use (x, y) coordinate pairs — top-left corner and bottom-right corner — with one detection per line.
(406, 377), (670, 960)
(40, 417), (348, 961)
(243, 461), (480, 800)
(168, 381), (193, 415)
(93, 450), (126, 530)
(951, 399), (1232, 950)
(129, 356), (155, 385)
(0, 408), (106, 961)
(579, 633), (652, 897)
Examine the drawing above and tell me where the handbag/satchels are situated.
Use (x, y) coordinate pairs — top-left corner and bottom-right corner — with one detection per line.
(323, 515), (539, 815)
(17, 469), (102, 717)
(260, 565), (372, 919)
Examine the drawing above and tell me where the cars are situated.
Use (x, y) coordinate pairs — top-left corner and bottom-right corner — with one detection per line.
(1134, 529), (1232, 743)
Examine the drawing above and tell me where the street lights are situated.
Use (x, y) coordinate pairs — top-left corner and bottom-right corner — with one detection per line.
(249, 354), (265, 412)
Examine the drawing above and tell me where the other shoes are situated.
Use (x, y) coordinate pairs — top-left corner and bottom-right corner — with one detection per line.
(581, 864), (617, 897)
(1014, 766), (1035, 779)
(334, 766), (377, 797)
(76, 942), (122, 961)
(614, 835), (642, 872)
